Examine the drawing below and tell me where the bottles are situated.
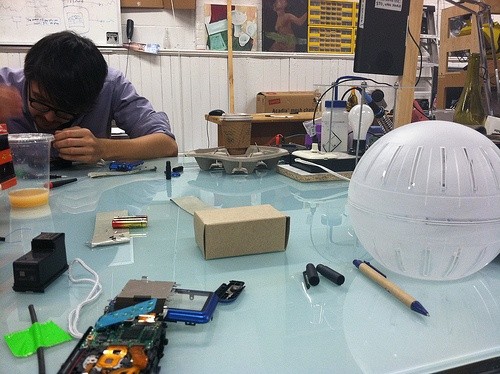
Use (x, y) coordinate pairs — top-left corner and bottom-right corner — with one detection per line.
(454, 52), (487, 131)
(321, 100), (349, 154)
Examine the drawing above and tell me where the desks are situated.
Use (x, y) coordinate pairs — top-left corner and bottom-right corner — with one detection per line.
(205, 114), (324, 154)
(0, 147), (500, 374)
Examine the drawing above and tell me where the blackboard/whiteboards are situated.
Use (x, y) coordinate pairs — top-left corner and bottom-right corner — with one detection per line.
(0, 0), (124, 46)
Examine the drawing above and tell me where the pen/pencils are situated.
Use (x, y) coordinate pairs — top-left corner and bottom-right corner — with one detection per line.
(50, 175), (67, 179)
(352, 258), (431, 317)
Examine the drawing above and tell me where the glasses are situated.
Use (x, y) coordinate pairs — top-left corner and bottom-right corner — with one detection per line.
(28, 79), (82, 120)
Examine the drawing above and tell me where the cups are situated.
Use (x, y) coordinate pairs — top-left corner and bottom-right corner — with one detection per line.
(434, 109), (454, 122)
(220, 113), (252, 155)
(8, 132), (55, 206)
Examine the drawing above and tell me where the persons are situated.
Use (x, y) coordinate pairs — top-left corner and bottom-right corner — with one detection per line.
(0, 30), (179, 169)
(266, 1), (309, 52)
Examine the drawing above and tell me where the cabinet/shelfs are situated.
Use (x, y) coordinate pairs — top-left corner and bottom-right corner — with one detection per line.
(435, 0), (500, 112)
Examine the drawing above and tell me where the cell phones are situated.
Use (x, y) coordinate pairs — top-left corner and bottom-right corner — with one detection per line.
(109, 288), (219, 323)
(109, 159), (144, 171)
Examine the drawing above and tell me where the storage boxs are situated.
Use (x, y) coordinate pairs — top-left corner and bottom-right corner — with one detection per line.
(255, 92), (321, 113)
(193, 204), (290, 261)
